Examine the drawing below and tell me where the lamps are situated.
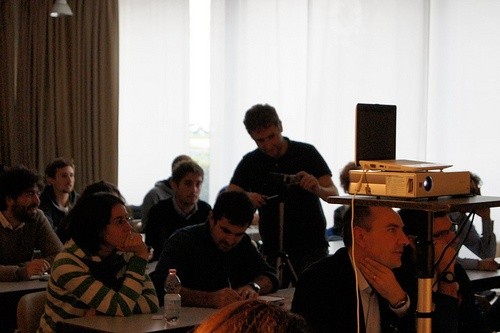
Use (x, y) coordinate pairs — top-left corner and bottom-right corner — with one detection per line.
(49, 0), (73, 18)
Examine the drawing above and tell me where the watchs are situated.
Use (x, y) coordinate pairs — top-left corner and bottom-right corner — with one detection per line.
(247, 282), (265, 296)
(394, 300), (406, 309)
(437, 271), (456, 283)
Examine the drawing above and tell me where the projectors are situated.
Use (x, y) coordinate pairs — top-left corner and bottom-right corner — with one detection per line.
(349, 170), (471, 197)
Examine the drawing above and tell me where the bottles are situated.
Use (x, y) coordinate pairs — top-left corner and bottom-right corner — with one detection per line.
(164, 269), (182, 326)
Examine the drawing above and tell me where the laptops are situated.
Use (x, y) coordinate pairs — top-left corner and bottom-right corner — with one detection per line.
(355, 103), (453, 171)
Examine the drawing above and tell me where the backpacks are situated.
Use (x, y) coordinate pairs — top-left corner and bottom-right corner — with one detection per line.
(193, 298), (288, 333)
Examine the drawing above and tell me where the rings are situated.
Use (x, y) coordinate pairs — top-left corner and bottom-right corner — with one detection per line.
(373, 275), (377, 280)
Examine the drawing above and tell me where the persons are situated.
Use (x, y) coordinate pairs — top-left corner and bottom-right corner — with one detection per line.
(145, 160), (212, 263)
(141, 156), (192, 227)
(0, 163), (62, 282)
(290, 205), (417, 333)
(36, 193), (160, 333)
(397, 175), (500, 333)
(55, 180), (122, 245)
(38, 157), (82, 235)
(333, 161), (357, 235)
(196, 298), (307, 333)
(228, 105), (339, 289)
(155, 190), (282, 309)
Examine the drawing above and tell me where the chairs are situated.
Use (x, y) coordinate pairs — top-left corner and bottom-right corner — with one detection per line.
(18, 292), (49, 333)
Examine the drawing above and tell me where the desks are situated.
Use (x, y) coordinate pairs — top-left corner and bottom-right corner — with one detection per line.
(327, 193), (500, 333)
(56, 306), (222, 333)
(0, 274), (47, 294)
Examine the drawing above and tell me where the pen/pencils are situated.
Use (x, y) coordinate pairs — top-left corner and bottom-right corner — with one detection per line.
(226, 278), (231, 289)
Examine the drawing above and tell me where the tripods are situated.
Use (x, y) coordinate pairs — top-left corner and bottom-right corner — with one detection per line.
(263, 192), (297, 287)
